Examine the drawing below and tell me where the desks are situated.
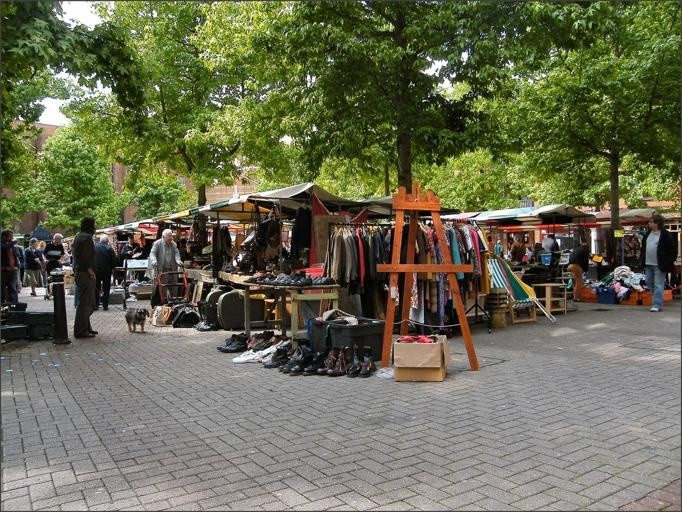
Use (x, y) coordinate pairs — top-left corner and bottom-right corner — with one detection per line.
(532, 283), (567, 316)
(240, 281), (340, 350)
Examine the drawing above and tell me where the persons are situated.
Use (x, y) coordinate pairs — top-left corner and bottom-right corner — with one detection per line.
(70, 216), (99, 338)
(1, 228), (72, 306)
(121, 234), (144, 259)
(509, 233), (526, 262)
(151, 229), (185, 311)
(94, 233), (117, 310)
(493, 239), (504, 259)
(638, 215), (677, 312)
(567, 242), (591, 302)
(542, 234), (559, 251)
(528, 243), (545, 263)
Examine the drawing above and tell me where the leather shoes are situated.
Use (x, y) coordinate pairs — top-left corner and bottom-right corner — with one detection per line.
(89, 330), (98, 334)
(242, 272), (335, 285)
(88, 333), (94, 337)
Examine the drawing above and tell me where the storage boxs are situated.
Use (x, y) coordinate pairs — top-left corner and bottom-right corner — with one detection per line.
(541, 253), (552, 265)
(390, 334), (450, 382)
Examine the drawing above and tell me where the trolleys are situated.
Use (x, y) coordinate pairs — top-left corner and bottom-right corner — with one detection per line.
(155, 265), (189, 304)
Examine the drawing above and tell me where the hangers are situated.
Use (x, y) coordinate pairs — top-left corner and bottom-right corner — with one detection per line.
(333, 217), (474, 233)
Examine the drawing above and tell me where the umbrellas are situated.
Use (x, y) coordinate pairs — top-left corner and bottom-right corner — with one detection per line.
(495, 255), (557, 325)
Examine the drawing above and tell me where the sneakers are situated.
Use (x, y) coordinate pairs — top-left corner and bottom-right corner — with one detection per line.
(650, 307), (663, 312)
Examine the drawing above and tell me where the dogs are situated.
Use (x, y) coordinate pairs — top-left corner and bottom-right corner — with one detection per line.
(125, 307), (150, 333)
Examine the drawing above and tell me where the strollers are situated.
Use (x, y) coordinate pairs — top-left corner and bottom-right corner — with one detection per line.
(42, 270), (53, 300)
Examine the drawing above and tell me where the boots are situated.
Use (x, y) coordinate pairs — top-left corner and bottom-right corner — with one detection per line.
(360, 346), (372, 377)
(316, 349), (338, 374)
(217, 332), (291, 368)
(303, 349), (325, 375)
(327, 350), (347, 377)
(199, 303), (218, 332)
(290, 353), (314, 375)
(283, 340), (311, 372)
(194, 301), (203, 330)
(348, 345), (361, 377)
(279, 338), (313, 373)
